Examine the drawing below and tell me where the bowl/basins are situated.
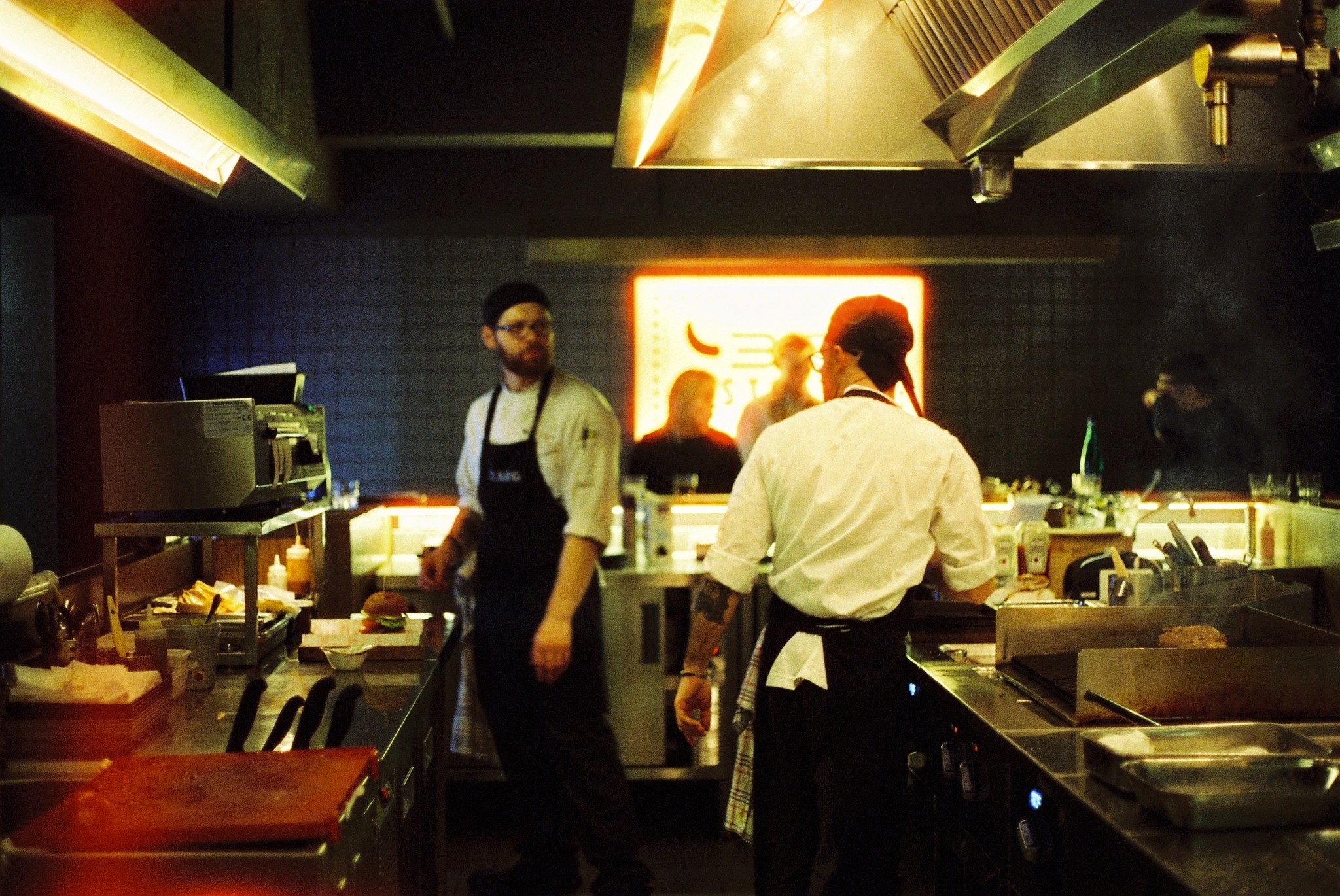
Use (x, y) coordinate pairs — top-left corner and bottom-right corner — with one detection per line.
(320, 644), (372, 671)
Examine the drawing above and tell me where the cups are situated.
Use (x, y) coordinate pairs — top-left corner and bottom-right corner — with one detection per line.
(979, 471), (1140, 579)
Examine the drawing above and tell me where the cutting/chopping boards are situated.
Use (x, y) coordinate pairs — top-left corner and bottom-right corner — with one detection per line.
(12, 746), (375, 848)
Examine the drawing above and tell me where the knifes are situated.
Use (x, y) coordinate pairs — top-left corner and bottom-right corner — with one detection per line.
(225, 677), (364, 754)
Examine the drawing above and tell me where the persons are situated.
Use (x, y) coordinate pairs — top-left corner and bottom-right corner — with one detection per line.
(628, 370), (743, 494)
(1142, 360), (1262, 491)
(419, 282), (657, 896)
(674, 296), (1000, 896)
(735, 335), (824, 463)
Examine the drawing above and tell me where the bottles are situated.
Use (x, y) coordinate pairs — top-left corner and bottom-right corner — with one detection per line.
(268, 554), (288, 590)
(286, 534), (311, 599)
(137, 603), (169, 673)
(1245, 472), (1323, 564)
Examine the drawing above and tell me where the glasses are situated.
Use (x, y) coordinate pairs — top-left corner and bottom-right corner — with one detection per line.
(808, 346), (861, 372)
(492, 320), (554, 331)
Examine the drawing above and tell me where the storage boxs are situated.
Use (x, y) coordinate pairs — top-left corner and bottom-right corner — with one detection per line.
(7, 669), (175, 754)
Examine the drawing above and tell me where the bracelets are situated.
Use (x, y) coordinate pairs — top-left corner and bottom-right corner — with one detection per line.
(444, 536), (464, 572)
(680, 670), (711, 678)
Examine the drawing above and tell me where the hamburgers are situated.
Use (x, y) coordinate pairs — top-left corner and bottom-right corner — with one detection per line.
(358, 591), (408, 634)
(1157, 625), (1228, 649)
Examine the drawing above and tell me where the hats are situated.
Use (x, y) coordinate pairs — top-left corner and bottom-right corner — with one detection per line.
(827, 294), (914, 379)
(484, 282), (550, 327)
(1158, 353), (1215, 387)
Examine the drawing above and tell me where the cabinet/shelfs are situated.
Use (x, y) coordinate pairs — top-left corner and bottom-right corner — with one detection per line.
(1, 609), (464, 894)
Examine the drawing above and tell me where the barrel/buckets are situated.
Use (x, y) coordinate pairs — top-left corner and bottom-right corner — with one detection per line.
(160, 617), (219, 691)
